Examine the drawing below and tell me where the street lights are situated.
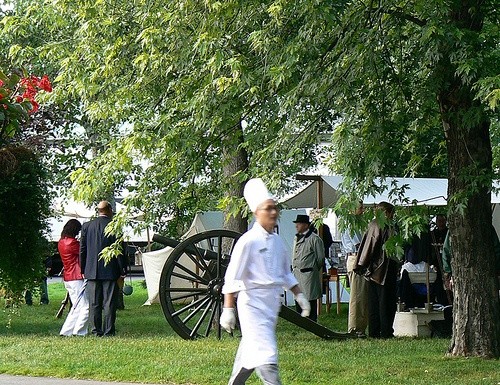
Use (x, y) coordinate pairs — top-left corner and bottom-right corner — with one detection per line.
(295, 174), (324, 240)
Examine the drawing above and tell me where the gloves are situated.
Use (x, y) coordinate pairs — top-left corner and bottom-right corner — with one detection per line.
(219, 307), (236, 334)
(295, 292), (311, 317)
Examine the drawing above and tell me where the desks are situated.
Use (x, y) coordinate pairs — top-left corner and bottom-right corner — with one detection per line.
(397, 271), (438, 312)
(392, 311), (444, 338)
(316, 273), (348, 317)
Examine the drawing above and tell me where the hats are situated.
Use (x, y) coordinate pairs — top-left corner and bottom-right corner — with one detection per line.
(292, 214), (312, 224)
(243, 177), (273, 212)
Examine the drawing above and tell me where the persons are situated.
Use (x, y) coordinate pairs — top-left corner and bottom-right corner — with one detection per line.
(80, 199), (127, 337)
(289, 199), (500, 339)
(25, 237), (51, 305)
(220, 175), (311, 385)
(58, 219), (90, 337)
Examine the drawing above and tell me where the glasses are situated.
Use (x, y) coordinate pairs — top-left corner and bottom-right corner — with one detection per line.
(256, 206), (277, 212)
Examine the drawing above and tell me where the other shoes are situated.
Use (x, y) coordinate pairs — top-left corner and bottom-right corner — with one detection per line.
(356, 331), (368, 338)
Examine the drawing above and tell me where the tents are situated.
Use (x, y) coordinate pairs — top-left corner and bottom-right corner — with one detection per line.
(141, 209), (350, 308)
(276, 178), (500, 241)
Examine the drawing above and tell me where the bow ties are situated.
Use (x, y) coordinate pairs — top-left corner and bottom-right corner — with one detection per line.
(295, 233), (304, 242)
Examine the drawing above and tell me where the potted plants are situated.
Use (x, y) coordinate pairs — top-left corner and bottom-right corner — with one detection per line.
(328, 257), (340, 275)
